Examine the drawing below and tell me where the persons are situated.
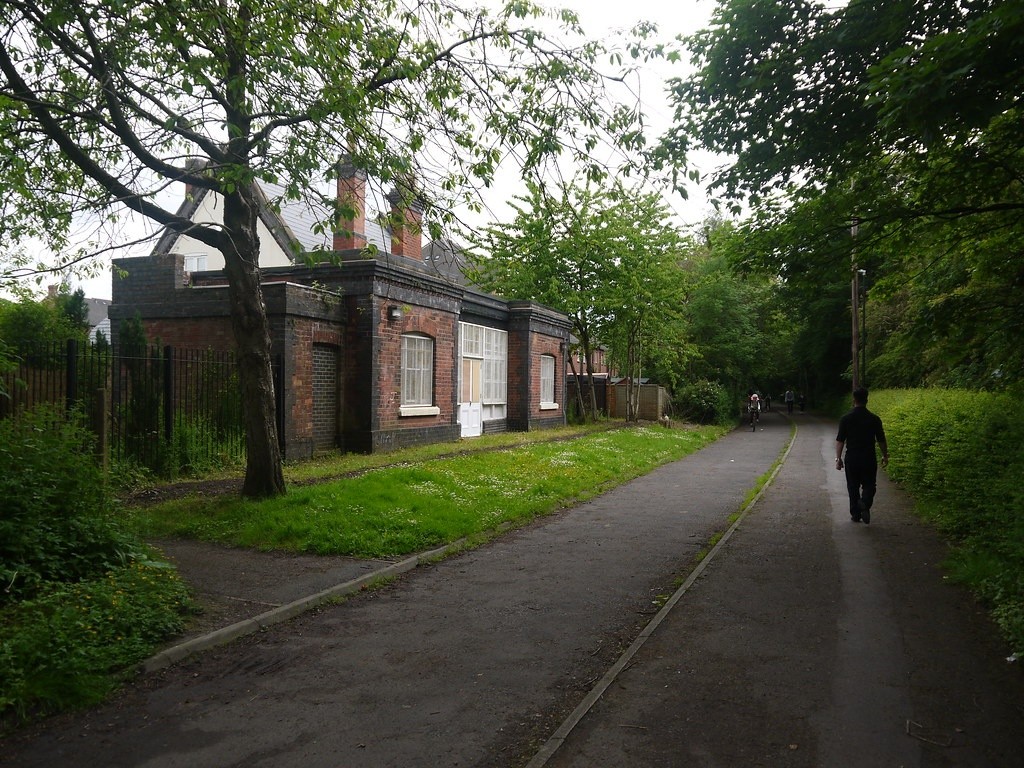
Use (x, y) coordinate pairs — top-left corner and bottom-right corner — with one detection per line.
(785, 388), (794, 415)
(761, 393), (771, 413)
(799, 391), (807, 415)
(834, 385), (889, 524)
(748, 394), (760, 425)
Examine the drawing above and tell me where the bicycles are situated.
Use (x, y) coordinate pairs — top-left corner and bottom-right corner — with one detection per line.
(786, 399), (794, 416)
(747, 409), (761, 432)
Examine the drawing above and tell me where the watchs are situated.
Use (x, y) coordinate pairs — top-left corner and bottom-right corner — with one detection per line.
(835, 458), (840, 461)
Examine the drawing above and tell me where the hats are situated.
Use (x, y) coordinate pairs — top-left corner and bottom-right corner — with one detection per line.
(750, 394), (759, 401)
